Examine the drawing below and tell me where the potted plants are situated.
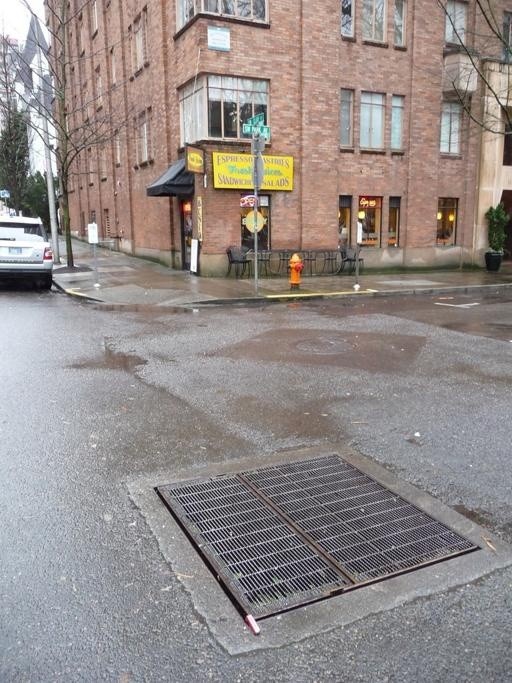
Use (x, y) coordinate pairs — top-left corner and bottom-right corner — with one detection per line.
(484, 202), (512, 272)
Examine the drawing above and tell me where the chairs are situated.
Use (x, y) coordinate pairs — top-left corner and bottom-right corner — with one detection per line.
(225, 245), (364, 280)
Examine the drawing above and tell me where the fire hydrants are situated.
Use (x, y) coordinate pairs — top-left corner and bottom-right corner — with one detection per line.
(287, 253), (304, 290)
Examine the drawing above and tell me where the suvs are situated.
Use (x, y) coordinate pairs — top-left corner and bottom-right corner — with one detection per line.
(0, 214), (55, 291)
